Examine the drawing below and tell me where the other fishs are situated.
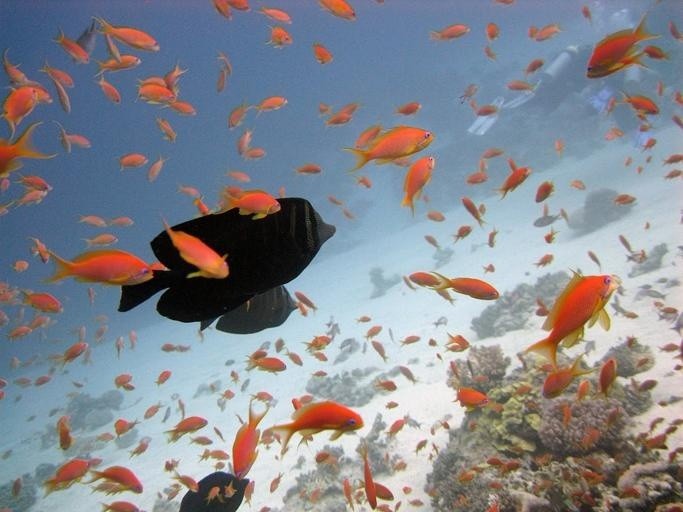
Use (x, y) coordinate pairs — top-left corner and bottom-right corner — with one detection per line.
(0, 0), (683, 512)
